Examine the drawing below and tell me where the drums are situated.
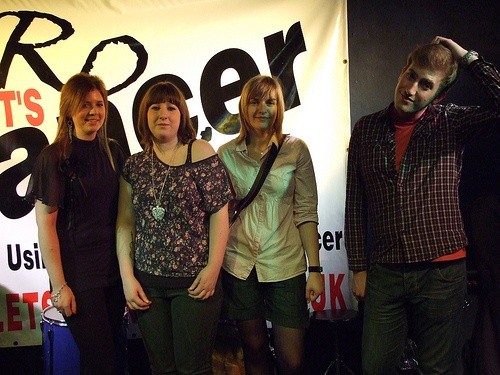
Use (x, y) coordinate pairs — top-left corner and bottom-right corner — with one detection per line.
(398, 335), (422, 375)
(40, 304), (130, 375)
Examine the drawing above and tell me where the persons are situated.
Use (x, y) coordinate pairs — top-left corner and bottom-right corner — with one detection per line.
(115, 80), (234, 375)
(31, 72), (126, 375)
(345, 37), (500, 375)
(218, 74), (325, 375)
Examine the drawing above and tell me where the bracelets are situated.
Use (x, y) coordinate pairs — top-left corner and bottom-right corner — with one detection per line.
(51, 282), (68, 302)
(309, 266), (323, 273)
(462, 50), (479, 65)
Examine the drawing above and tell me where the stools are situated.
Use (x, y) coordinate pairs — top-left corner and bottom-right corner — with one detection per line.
(314, 309), (358, 375)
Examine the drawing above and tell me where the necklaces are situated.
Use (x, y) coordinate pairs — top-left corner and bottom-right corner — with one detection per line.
(246, 140), (268, 156)
(151, 141), (181, 222)
